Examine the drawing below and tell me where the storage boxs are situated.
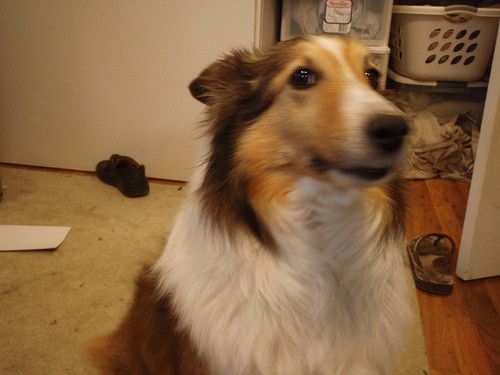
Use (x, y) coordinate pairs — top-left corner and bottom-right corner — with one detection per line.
(391, 3), (499, 83)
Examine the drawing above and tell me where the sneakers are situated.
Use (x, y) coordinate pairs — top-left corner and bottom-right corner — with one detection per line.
(96, 154), (150, 197)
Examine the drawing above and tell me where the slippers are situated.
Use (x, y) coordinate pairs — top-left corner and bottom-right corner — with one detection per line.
(408, 231), (456, 295)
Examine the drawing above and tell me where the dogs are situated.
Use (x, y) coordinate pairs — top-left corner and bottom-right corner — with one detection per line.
(86, 32), (430, 375)
